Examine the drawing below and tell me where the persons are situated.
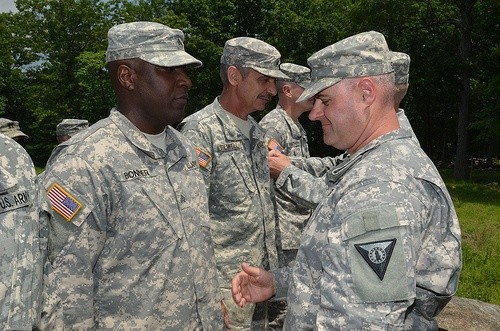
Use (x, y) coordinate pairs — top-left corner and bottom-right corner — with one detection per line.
(55, 118), (90, 146)
(0, 133), (44, 331)
(0, 116), (29, 147)
(266, 51), (420, 213)
(231, 31), (462, 331)
(39, 22), (230, 331)
(255, 61), (316, 331)
(174, 36), (291, 331)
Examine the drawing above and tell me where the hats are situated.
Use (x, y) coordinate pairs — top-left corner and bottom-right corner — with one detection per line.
(277, 63), (311, 89)
(295, 31), (395, 103)
(0, 118), (29, 138)
(221, 37), (291, 79)
(57, 119), (89, 137)
(106, 22), (202, 68)
(390, 51), (410, 85)
